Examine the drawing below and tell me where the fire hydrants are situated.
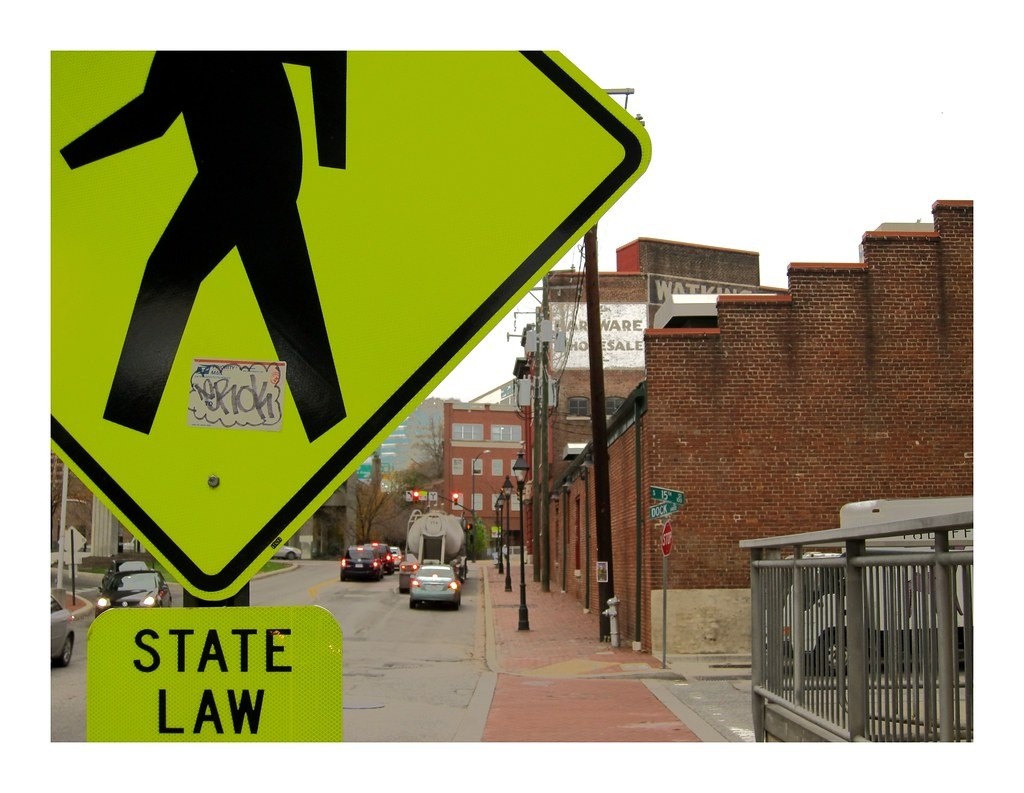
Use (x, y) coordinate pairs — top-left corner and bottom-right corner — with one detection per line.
(602, 596), (621, 648)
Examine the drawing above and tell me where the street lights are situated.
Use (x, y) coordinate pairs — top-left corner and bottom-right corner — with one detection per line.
(472, 450), (490, 563)
(494, 475), (514, 592)
(512, 453), (531, 630)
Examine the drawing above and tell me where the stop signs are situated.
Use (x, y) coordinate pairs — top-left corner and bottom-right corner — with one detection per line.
(660, 520), (674, 557)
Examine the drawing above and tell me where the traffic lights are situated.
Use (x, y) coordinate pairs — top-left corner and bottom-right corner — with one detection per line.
(453, 494), (459, 505)
(467, 524), (472, 530)
(413, 491), (418, 500)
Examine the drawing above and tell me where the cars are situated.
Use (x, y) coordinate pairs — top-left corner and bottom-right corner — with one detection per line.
(273, 545), (302, 560)
(51, 595), (75, 667)
(93, 559), (173, 618)
(340, 543), (404, 582)
(409, 565), (462, 610)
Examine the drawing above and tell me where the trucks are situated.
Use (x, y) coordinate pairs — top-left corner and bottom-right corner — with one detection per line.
(761, 496), (974, 675)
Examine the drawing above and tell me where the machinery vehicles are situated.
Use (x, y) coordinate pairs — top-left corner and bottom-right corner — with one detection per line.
(399, 509), (467, 593)
